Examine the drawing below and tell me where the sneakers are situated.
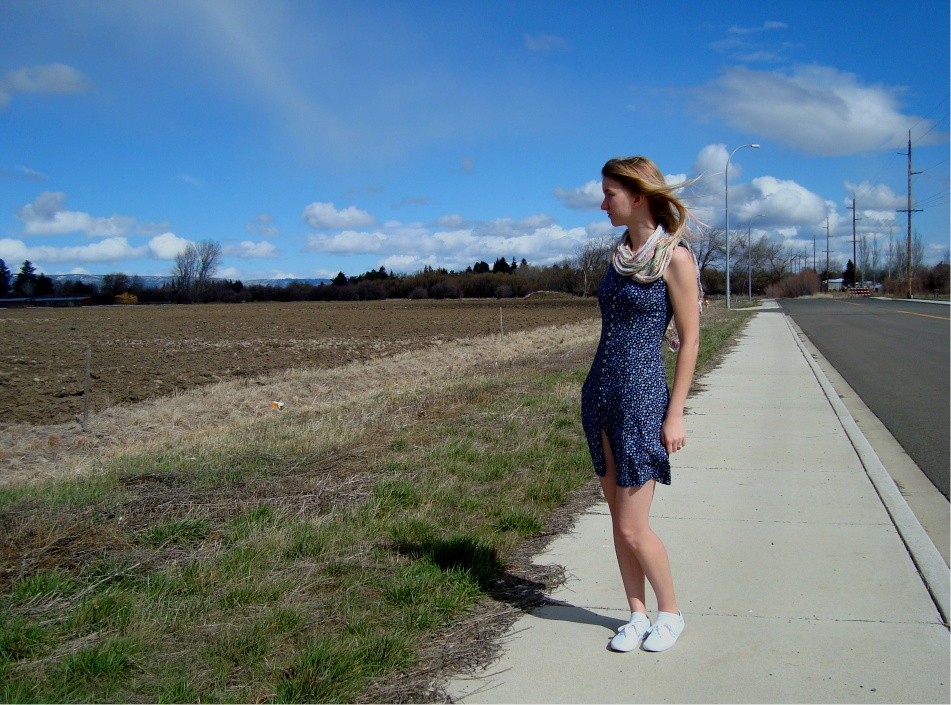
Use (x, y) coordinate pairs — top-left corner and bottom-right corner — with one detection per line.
(642, 610), (685, 651)
(610, 611), (653, 652)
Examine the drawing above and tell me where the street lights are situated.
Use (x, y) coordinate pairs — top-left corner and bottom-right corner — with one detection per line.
(726, 144), (760, 308)
(748, 214), (765, 300)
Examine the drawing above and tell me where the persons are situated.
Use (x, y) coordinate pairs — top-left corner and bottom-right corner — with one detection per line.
(580, 158), (700, 653)
